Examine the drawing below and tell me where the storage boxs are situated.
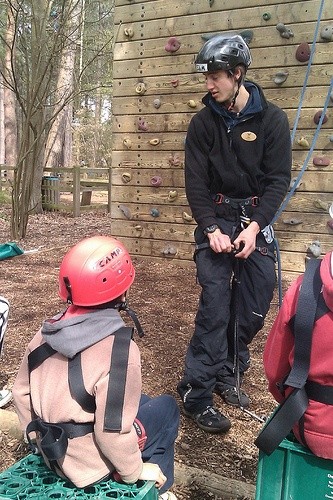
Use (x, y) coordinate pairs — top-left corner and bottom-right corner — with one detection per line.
(256, 404), (333, 500)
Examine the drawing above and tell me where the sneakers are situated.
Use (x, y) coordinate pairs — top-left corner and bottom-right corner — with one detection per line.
(213, 386), (249, 408)
(183, 404), (231, 432)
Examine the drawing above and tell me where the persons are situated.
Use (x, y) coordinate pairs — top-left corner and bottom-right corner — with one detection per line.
(0, 297), (13, 408)
(12, 236), (179, 500)
(263, 249), (333, 461)
(177, 33), (292, 433)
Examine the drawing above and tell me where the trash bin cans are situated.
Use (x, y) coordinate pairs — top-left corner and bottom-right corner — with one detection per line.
(42, 176), (59, 212)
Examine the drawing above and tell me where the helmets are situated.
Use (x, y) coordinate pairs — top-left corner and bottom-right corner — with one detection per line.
(58, 235), (135, 307)
(195, 32), (252, 73)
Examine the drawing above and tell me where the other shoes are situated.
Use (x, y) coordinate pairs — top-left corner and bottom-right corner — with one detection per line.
(0, 390), (13, 408)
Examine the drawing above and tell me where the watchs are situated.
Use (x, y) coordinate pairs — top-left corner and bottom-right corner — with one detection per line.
(203, 224), (220, 235)
